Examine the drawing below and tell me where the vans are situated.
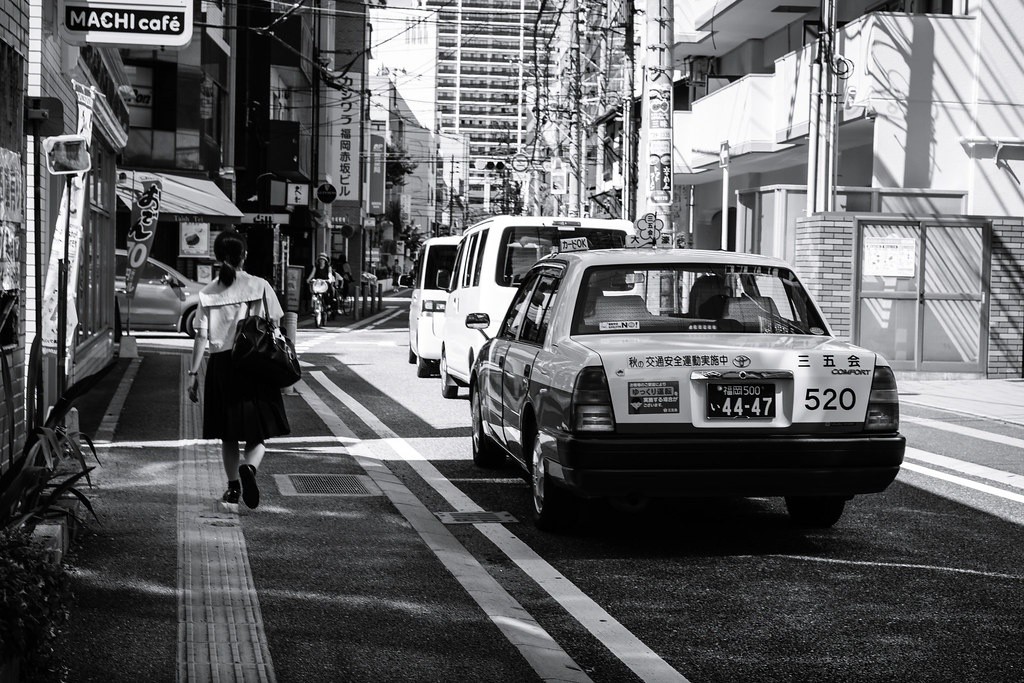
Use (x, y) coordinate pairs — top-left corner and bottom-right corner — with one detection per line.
(397, 236), (462, 377)
(439, 217), (636, 398)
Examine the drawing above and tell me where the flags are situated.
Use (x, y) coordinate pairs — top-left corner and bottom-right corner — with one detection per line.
(124, 177), (163, 299)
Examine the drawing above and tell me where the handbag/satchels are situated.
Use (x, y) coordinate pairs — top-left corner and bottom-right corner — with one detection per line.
(230, 297), (302, 388)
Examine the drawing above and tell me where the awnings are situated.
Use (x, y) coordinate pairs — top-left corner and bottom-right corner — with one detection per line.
(115, 169), (244, 225)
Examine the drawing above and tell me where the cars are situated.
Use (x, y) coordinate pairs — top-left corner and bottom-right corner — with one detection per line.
(116, 248), (206, 338)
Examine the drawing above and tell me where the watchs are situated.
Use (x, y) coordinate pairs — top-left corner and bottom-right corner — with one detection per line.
(187, 370), (198, 375)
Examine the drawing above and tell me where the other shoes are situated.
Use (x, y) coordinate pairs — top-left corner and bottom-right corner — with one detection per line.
(239, 464), (260, 509)
(222, 487), (240, 504)
(393, 289), (399, 292)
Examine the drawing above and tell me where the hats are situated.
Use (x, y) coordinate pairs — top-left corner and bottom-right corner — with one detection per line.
(318, 252), (328, 261)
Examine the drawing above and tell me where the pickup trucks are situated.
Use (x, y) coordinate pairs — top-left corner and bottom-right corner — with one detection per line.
(465, 248), (907, 529)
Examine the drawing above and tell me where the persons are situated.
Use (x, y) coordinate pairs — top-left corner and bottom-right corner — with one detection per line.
(186, 229), (285, 512)
(333, 255), (354, 299)
(307, 253), (335, 311)
(392, 258), (402, 292)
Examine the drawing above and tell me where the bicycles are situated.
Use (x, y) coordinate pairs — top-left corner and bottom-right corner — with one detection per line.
(334, 288), (352, 315)
(309, 278), (331, 326)
